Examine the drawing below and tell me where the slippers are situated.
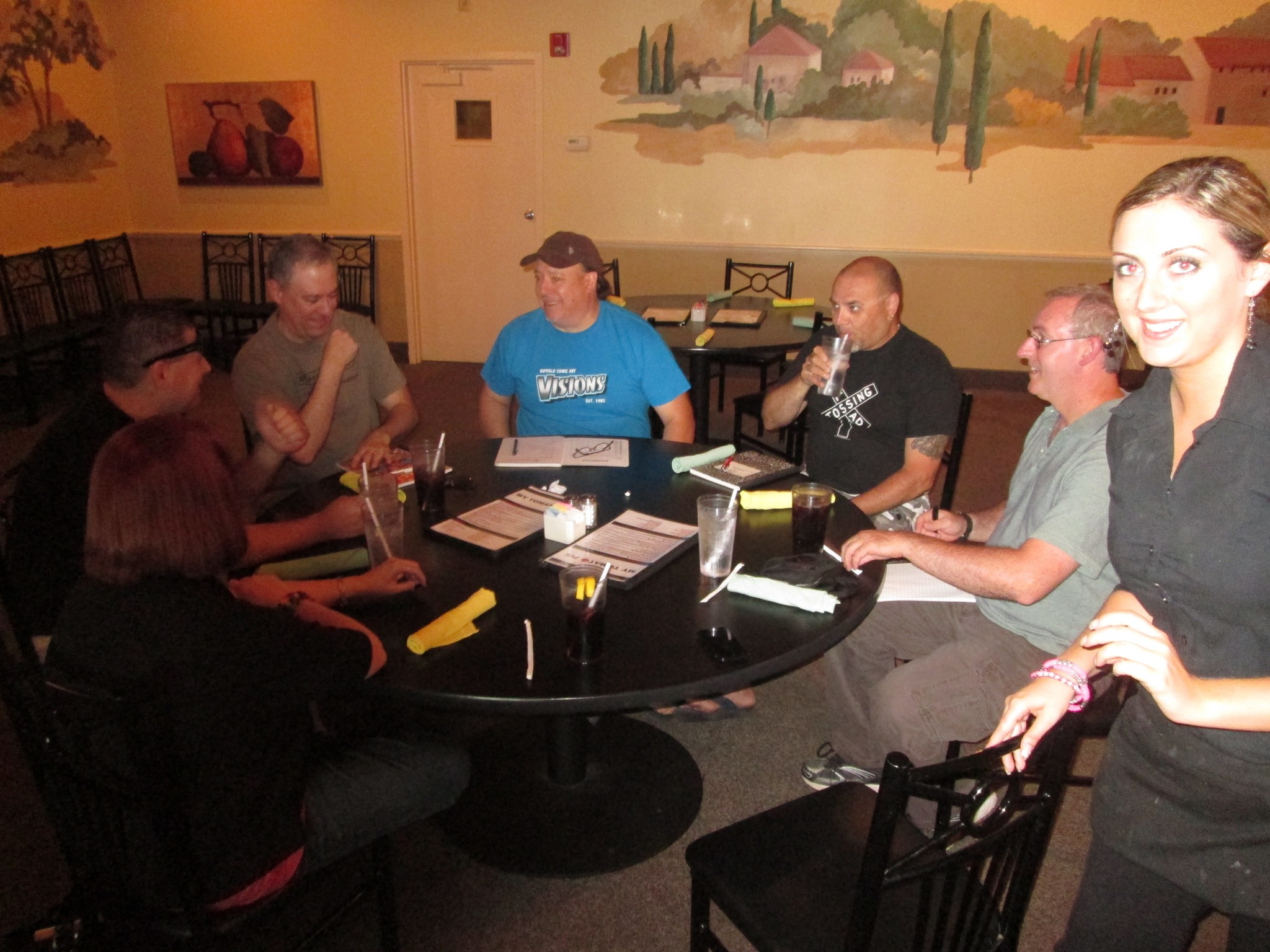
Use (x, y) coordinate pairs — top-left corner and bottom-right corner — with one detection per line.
(650, 695), (739, 723)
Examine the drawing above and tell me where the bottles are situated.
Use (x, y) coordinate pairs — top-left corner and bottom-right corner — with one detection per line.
(565, 495), (583, 511)
(580, 493), (597, 530)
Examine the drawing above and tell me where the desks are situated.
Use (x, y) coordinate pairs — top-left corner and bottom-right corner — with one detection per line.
(223, 431), (889, 884)
(621, 292), (834, 446)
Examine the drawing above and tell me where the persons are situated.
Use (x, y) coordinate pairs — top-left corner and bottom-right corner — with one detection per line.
(985, 157), (1270, 952)
(654, 256), (962, 721)
(480, 232), (695, 444)
(39, 414), (469, 924)
(800, 287), (1132, 793)
(230, 233), (420, 490)
(10, 303), (364, 662)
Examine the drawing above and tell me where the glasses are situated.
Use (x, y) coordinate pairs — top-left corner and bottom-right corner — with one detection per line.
(1026, 328), (1099, 351)
(144, 337), (205, 368)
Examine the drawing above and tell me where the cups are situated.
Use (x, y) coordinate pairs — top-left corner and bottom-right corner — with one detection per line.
(409, 442), (446, 523)
(791, 481), (833, 564)
(696, 495), (739, 581)
(357, 471), (398, 499)
(816, 334), (854, 398)
(360, 499), (405, 580)
(558, 564), (609, 668)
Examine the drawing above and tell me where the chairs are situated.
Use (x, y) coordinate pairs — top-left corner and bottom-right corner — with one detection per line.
(319, 229), (378, 329)
(0, 247), (91, 402)
(44, 240), (124, 329)
(677, 720), (1092, 952)
(730, 310), (834, 465)
(89, 231), (194, 339)
(928, 662), (1129, 840)
(0, 279), (68, 428)
(0, 621), (404, 952)
(599, 255), (623, 299)
(714, 257), (797, 415)
(939, 391), (974, 511)
(174, 229), (256, 362)
(230, 231), (282, 349)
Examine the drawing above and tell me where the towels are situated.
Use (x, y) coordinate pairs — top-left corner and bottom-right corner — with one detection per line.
(695, 328), (714, 346)
(607, 295), (626, 307)
(672, 443), (737, 473)
(261, 547), (370, 579)
(707, 290), (732, 302)
(740, 488), (835, 510)
(792, 317), (823, 329)
(338, 471), (409, 503)
(725, 573), (841, 615)
(772, 297), (815, 308)
(406, 586), (497, 655)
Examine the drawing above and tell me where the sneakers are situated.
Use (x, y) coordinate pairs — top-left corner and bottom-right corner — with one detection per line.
(800, 742), (883, 793)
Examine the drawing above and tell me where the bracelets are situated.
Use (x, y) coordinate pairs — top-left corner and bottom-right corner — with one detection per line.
(338, 577), (347, 607)
(960, 512), (973, 541)
(279, 591), (308, 621)
(1030, 659), (1090, 712)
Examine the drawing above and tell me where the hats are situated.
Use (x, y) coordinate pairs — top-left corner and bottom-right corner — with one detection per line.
(520, 231), (602, 274)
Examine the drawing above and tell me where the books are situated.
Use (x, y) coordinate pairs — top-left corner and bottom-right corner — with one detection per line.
(494, 436), (629, 467)
(543, 509), (699, 589)
(430, 484), (567, 550)
(689, 450), (800, 491)
(876, 563), (977, 604)
